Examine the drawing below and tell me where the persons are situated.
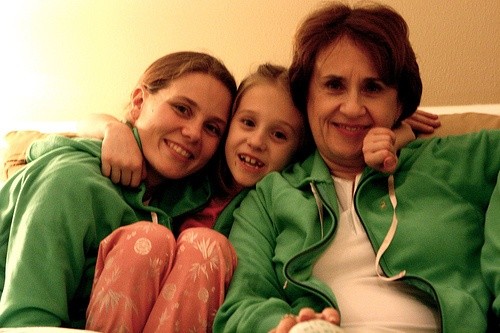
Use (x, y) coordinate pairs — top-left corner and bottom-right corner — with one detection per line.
(1, 52), (440, 333)
(65, 65), (415, 333)
(205, 2), (500, 332)
